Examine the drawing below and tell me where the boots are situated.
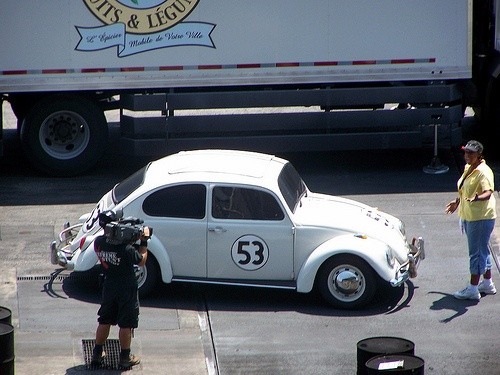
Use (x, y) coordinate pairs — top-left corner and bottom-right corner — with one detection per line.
(91, 349), (104, 365)
(118, 354), (140, 369)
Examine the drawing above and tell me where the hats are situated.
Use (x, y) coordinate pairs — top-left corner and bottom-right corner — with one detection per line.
(99, 210), (117, 227)
(462, 140), (483, 154)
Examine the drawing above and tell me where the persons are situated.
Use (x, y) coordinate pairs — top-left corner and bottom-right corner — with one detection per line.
(446, 141), (497, 300)
(91, 210), (150, 369)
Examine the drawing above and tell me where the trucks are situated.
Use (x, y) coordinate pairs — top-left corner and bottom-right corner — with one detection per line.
(1, 0), (500, 180)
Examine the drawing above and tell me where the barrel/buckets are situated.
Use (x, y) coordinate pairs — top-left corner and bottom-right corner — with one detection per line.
(0, 306), (13, 325)
(357, 336), (415, 375)
(365, 354), (425, 375)
(0, 322), (15, 375)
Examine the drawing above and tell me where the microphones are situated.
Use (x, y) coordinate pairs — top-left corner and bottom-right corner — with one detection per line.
(136, 220), (145, 224)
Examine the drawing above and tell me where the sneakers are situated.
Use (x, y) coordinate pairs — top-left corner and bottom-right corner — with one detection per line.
(478, 280), (497, 294)
(453, 279), (481, 299)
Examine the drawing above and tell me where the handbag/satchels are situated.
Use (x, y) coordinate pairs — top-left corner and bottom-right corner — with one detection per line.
(459, 216), (465, 235)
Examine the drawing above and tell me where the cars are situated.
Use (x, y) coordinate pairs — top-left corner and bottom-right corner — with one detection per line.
(50, 149), (426, 313)
